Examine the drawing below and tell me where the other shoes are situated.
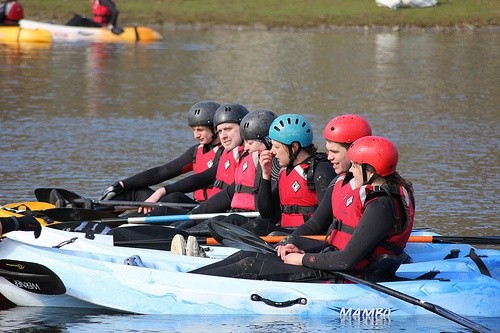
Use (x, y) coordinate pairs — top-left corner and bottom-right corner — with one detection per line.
(124, 255), (145, 267)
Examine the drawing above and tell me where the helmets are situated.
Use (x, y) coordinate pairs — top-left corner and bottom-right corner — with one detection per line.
(323, 114), (372, 144)
(346, 136), (399, 177)
(269, 114), (313, 148)
(213, 103), (249, 130)
(239, 109), (278, 142)
(187, 100), (221, 126)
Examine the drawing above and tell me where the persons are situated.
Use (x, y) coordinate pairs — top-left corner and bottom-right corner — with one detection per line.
(0, 215), (42, 238)
(66, 0), (118, 30)
(0, 0), (24, 26)
(49, 101), (415, 284)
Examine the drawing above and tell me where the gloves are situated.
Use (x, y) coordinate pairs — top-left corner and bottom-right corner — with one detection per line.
(11, 214), (42, 239)
(97, 182), (125, 201)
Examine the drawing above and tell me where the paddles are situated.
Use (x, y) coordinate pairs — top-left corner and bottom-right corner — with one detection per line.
(34, 188), (199, 208)
(408, 235), (500, 246)
(42, 208), (260, 224)
(73, 12), (124, 35)
(113, 225), (329, 252)
(331, 273), (491, 332)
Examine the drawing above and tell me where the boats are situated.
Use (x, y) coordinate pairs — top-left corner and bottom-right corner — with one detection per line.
(0, 25), (54, 51)
(17, 19), (164, 46)
(0, 199), (500, 316)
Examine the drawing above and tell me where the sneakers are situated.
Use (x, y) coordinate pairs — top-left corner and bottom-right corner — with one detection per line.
(85, 199), (95, 209)
(49, 189), (75, 208)
(186, 236), (207, 258)
(171, 234), (186, 255)
